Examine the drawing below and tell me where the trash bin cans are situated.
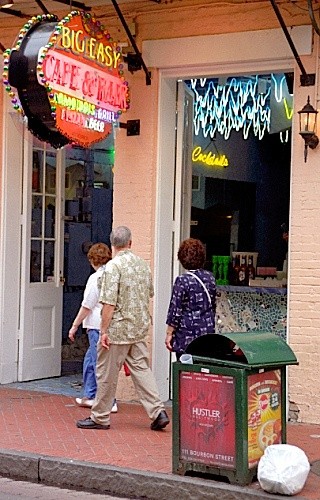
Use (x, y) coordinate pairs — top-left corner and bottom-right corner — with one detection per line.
(172, 332), (299, 488)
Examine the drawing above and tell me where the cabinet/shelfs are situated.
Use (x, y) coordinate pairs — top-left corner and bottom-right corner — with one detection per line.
(30, 144), (116, 339)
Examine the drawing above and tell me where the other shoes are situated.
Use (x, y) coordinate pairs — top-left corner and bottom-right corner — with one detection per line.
(75, 397), (94, 408)
(110, 403), (118, 412)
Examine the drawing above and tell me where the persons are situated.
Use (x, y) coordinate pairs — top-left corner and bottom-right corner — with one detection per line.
(165, 238), (217, 362)
(69, 243), (118, 413)
(76, 225), (170, 429)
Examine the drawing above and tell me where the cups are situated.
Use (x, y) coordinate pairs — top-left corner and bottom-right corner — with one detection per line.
(181, 354), (194, 365)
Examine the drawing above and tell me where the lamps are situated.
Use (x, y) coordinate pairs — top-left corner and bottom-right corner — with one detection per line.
(298, 94), (319, 163)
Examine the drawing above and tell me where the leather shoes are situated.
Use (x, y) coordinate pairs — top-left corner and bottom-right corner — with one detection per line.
(150, 410), (170, 430)
(77, 417), (110, 429)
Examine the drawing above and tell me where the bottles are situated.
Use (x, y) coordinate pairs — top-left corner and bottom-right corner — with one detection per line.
(233, 253), (256, 286)
